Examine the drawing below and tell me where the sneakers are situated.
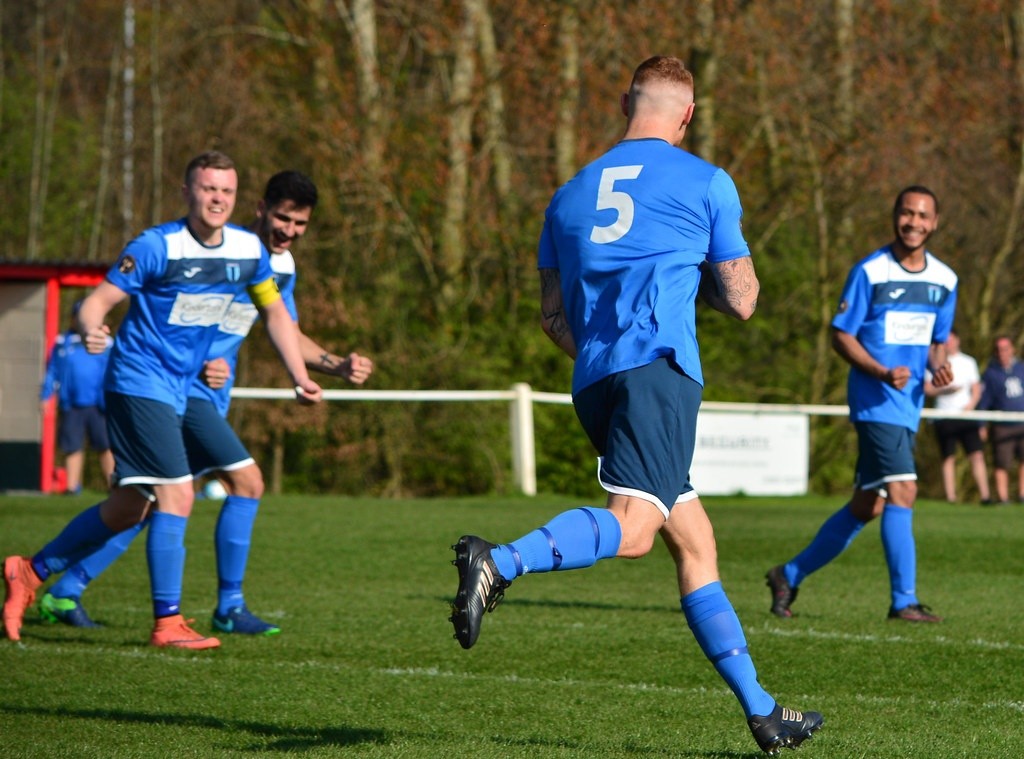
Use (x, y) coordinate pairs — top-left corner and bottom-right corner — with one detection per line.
(211, 603), (282, 637)
(448, 534), (512, 650)
(148, 615), (221, 650)
(2, 555), (44, 640)
(748, 703), (824, 758)
(37, 587), (106, 631)
(765, 565), (800, 617)
(887, 603), (944, 622)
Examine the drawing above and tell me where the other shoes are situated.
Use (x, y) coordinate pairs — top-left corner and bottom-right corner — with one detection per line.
(62, 486), (83, 496)
(980, 498), (992, 507)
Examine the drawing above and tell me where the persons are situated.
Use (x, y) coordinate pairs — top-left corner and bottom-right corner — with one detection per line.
(448, 55), (823, 755)
(762, 185), (959, 623)
(924, 330), (1024, 507)
(0, 150), (373, 650)
